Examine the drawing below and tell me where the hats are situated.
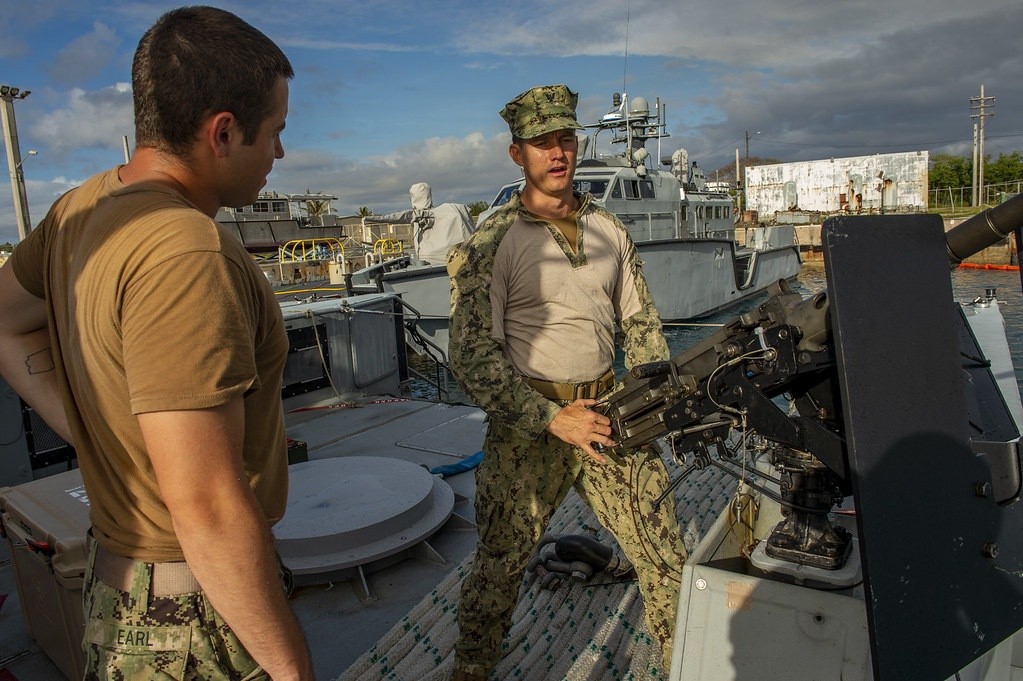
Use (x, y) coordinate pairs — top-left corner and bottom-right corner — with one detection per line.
(499, 84), (585, 139)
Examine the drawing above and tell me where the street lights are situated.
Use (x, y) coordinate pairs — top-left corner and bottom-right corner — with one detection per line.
(743, 130), (762, 167)
(0, 84), (39, 242)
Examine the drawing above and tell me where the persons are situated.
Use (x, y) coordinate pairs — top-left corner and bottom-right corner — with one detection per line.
(448, 86), (689, 681)
(0, 7), (323, 681)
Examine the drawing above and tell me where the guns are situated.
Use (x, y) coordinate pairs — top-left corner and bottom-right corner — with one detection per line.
(577, 192), (1023, 571)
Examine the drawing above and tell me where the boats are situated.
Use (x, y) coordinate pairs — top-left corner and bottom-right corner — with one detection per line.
(340, 2), (804, 366)
(216, 189), (348, 252)
